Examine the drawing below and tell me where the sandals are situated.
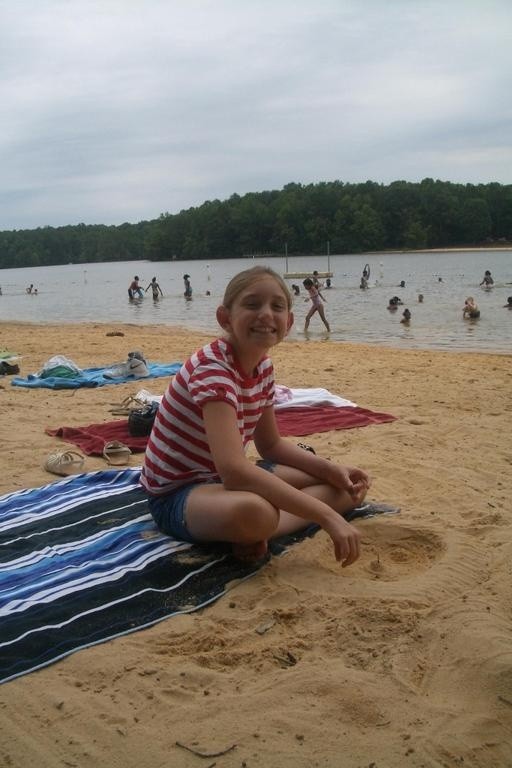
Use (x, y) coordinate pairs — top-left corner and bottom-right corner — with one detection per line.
(102, 440), (131, 466)
(45, 451), (86, 475)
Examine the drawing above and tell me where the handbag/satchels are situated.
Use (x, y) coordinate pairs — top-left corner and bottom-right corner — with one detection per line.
(128, 401), (160, 438)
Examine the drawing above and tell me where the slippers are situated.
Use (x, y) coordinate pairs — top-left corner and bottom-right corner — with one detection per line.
(109, 396), (150, 416)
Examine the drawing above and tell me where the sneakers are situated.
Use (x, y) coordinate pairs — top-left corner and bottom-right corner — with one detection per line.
(102, 358), (150, 379)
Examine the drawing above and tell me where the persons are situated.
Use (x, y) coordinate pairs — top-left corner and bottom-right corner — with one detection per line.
(387, 270), (512, 325)
(360, 263), (371, 289)
(183, 274), (192, 300)
(26, 285), (33, 294)
(129, 276), (163, 300)
(303, 278), (330, 334)
(293, 284), (299, 294)
(33, 289), (37, 294)
(324, 279), (333, 289)
(138, 267), (371, 568)
(313, 270), (326, 301)
